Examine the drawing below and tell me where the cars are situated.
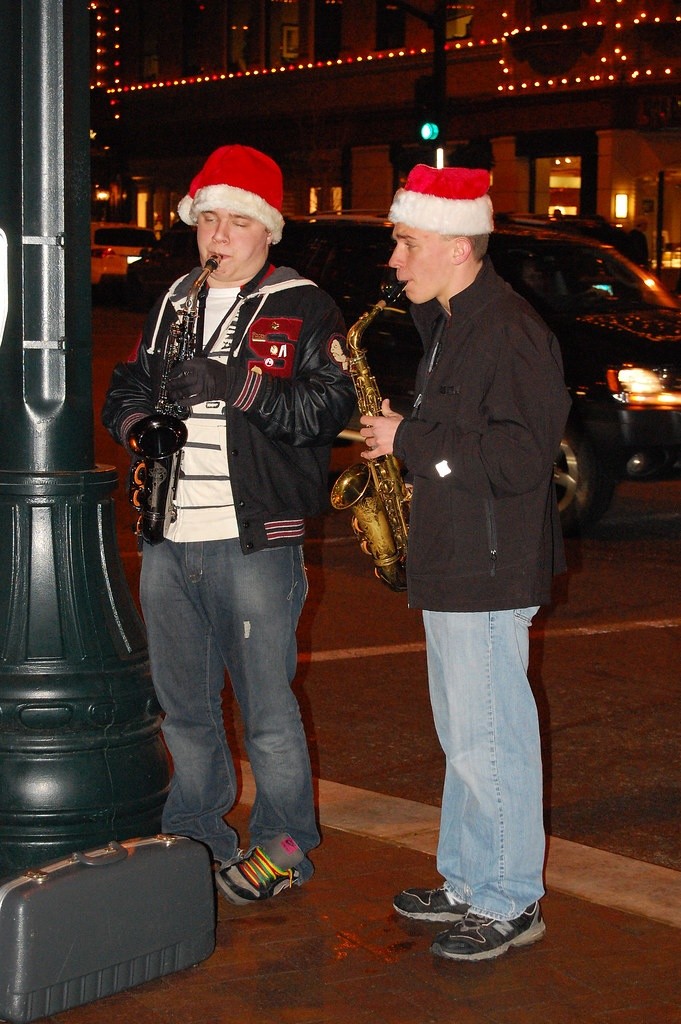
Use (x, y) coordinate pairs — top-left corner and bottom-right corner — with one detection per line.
(90, 220), (159, 302)
(121, 210), (681, 534)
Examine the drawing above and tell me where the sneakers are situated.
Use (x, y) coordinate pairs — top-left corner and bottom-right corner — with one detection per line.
(392, 880), (472, 924)
(428, 900), (546, 960)
(213, 835), (313, 906)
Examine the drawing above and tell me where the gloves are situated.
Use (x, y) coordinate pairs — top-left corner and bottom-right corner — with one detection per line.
(164, 356), (269, 417)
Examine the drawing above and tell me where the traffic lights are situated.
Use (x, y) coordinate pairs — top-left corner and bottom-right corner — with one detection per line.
(412, 74), (444, 147)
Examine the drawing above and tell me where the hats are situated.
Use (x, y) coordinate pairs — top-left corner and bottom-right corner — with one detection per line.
(176, 143), (286, 244)
(386, 163), (495, 236)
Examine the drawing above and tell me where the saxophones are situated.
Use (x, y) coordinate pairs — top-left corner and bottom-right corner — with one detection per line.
(330, 277), (413, 593)
(127, 254), (224, 547)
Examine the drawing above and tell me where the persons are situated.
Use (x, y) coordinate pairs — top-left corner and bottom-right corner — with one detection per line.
(359, 164), (574, 964)
(625, 218), (650, 267)
(100, 144), (357, 905)
